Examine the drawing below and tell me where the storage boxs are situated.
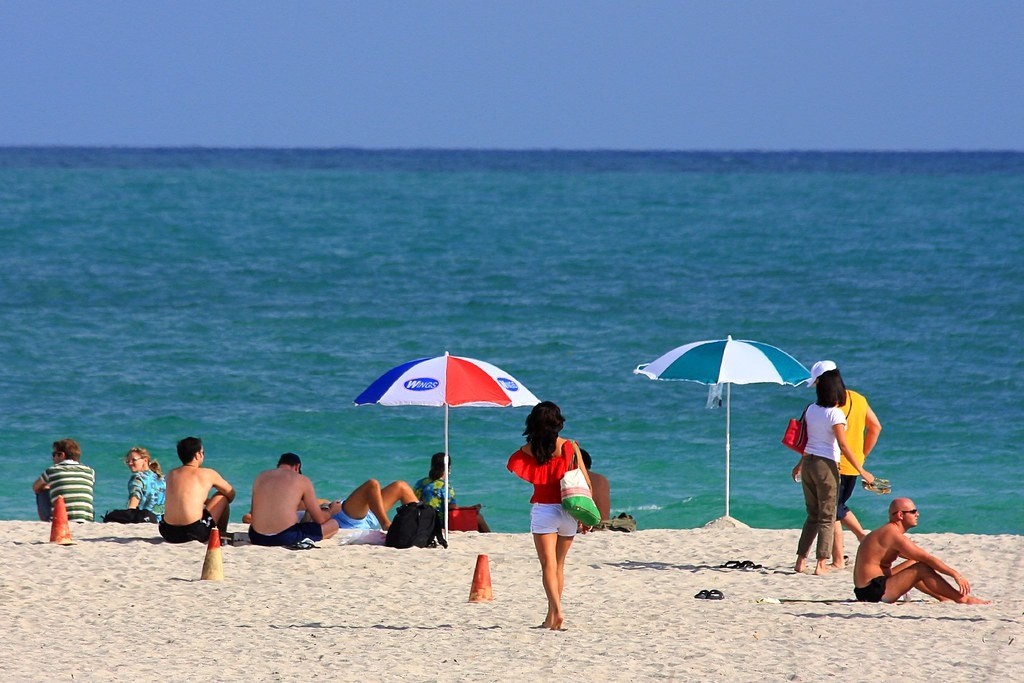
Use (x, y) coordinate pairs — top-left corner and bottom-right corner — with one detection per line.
(449, 509), (478, 531)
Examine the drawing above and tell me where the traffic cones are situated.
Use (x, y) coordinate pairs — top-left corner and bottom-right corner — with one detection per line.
(466, 554), (494, 603)
(48, 496), (79, 546)
(200, 529), (224, 581)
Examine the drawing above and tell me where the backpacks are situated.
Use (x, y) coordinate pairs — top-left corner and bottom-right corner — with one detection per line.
(99, 508), (158, 523)
(383, 501), (448, 550)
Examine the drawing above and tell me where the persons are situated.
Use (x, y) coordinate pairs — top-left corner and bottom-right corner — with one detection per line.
(852, 497), (991, 605)
(159, 437), (235, 543)
(33, 439), (95, 524)
(125, 447), (166, 522)
(791, 360), (881, 575)
(579, 448), (611, 522)
(413, 453), (491, 532)
(507, 401), (593, 630)
(242, 453), (421, 547)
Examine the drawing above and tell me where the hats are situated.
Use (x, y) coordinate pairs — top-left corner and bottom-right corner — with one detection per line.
(277, 453), (302, 473)
(806, 360), (837, 388)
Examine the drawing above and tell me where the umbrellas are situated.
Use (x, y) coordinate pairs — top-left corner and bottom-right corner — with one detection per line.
(352, 352), (542, 551)
(633, 333), (814, 528)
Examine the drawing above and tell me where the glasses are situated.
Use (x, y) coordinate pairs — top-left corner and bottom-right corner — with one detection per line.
(892, 508), (917, 516)
(125, 457), (145, 464)
(52, 451), (61, 457)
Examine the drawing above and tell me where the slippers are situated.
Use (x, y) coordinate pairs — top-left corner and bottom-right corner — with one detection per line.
(861, 477), (891, 495)
(722, 560), (762, 568)
(695, 590), (723, 600)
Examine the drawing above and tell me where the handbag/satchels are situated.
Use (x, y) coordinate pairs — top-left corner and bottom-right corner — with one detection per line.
(560, 439), (601, 526)
(781, 403), (814, 455)
